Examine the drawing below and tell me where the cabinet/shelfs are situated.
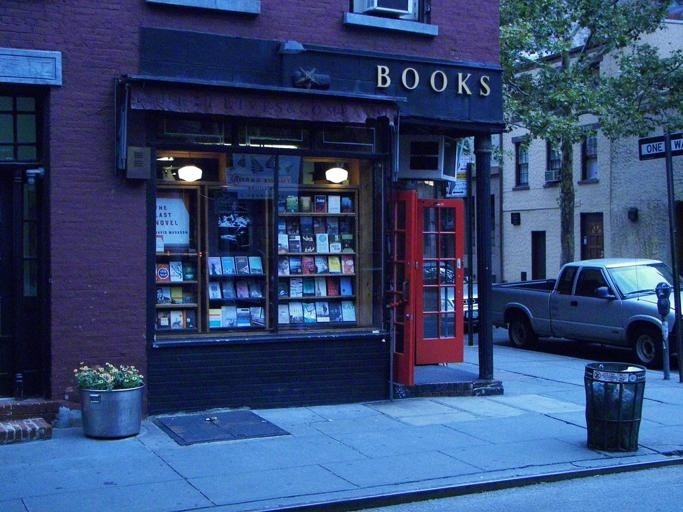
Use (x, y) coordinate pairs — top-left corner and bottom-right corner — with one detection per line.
(157, 151), (358, 329)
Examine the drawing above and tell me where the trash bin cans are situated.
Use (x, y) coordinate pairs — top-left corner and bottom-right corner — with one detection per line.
(585, 363), (645, 454)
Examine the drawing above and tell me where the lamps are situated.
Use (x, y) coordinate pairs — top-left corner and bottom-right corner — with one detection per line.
(278, 40), (306, 55)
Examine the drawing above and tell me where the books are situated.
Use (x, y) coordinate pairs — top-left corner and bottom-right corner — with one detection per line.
(155, 254), (267, 334)
(277, 192), (357, 323)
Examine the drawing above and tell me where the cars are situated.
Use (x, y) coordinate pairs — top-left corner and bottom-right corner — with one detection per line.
(422, 258), (486, 333)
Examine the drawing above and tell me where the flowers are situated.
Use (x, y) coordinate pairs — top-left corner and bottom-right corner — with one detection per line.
(70, 361), (144, 390)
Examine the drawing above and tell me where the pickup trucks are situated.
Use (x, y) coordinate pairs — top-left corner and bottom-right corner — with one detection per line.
(486, 255), (683, 370)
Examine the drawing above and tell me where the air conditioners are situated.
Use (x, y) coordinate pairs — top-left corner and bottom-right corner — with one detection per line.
(398, 135), (459, 182)
(361, 0), (413, 16)
